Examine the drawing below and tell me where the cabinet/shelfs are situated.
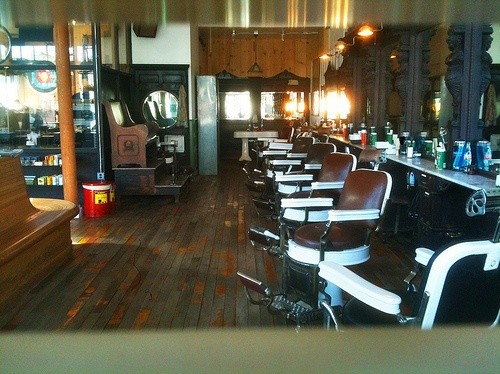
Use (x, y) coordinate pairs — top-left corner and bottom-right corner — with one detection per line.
(0, 63), (101, 205)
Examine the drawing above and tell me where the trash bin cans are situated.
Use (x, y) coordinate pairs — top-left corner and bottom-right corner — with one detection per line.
(82, 181), (112, 218)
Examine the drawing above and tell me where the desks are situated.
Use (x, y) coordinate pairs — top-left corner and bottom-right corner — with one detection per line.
(310, 130), (500, 244)
(233, 131), (278, 162)
(1, 129), (96, 149)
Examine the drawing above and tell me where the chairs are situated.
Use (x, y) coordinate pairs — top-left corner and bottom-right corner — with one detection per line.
(237, 137), (500, 329)
(104, 99), (158, 169)
(145, 96), (174, 127)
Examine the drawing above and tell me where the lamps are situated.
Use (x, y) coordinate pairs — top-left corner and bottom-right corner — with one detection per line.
(319, 21), (383, 59)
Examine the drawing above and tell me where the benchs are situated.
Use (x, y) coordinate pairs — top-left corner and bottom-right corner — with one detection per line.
(0, 156), (79, 302)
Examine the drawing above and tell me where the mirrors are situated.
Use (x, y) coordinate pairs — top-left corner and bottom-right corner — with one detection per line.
(140, 88), (181, 129)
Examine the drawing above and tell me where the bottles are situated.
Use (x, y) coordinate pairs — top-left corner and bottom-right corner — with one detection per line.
(342, 123), (492, 175)
